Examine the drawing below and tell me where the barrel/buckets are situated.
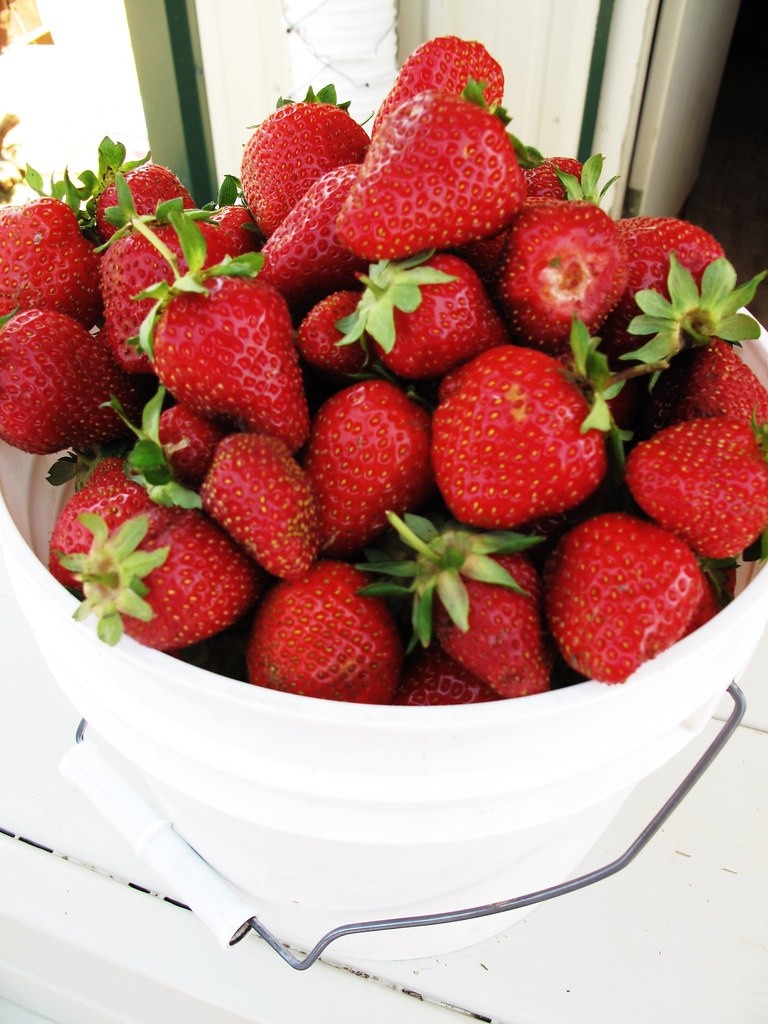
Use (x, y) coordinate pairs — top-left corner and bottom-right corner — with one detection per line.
(0, 298), (768, 972)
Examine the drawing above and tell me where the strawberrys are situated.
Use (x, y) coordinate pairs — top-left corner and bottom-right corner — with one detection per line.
(0, 36), (768, 706)
